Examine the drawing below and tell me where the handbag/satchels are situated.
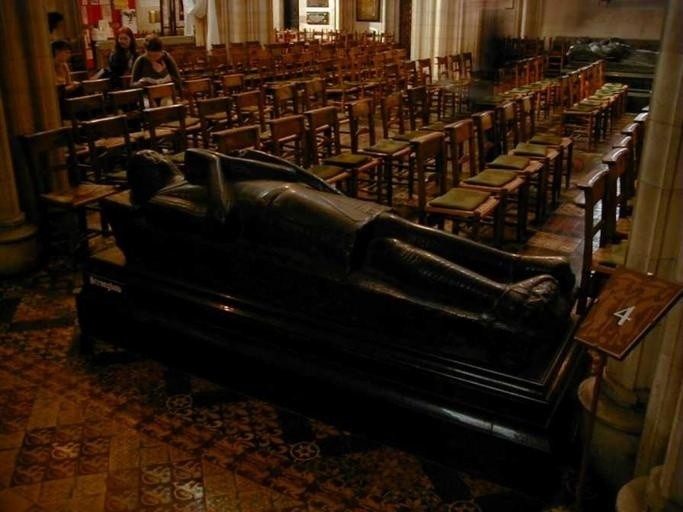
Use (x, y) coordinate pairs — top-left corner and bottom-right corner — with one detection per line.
(89, 66), (122, 89)
(104, 89), (137, 112)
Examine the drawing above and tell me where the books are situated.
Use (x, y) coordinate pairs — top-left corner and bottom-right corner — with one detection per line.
(140, 72), (172, 86)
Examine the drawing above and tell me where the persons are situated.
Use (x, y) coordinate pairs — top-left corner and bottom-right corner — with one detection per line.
(49, 39), (87, 125)
(127, 34), (185, 109)
(126, 145), (575, 322)
(574, 35), (660, 69)
(45, 11), (67, 39)
(109, 26), (143, 133)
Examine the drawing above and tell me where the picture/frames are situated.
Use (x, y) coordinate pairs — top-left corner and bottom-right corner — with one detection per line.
(306, 1), (381, 24)
